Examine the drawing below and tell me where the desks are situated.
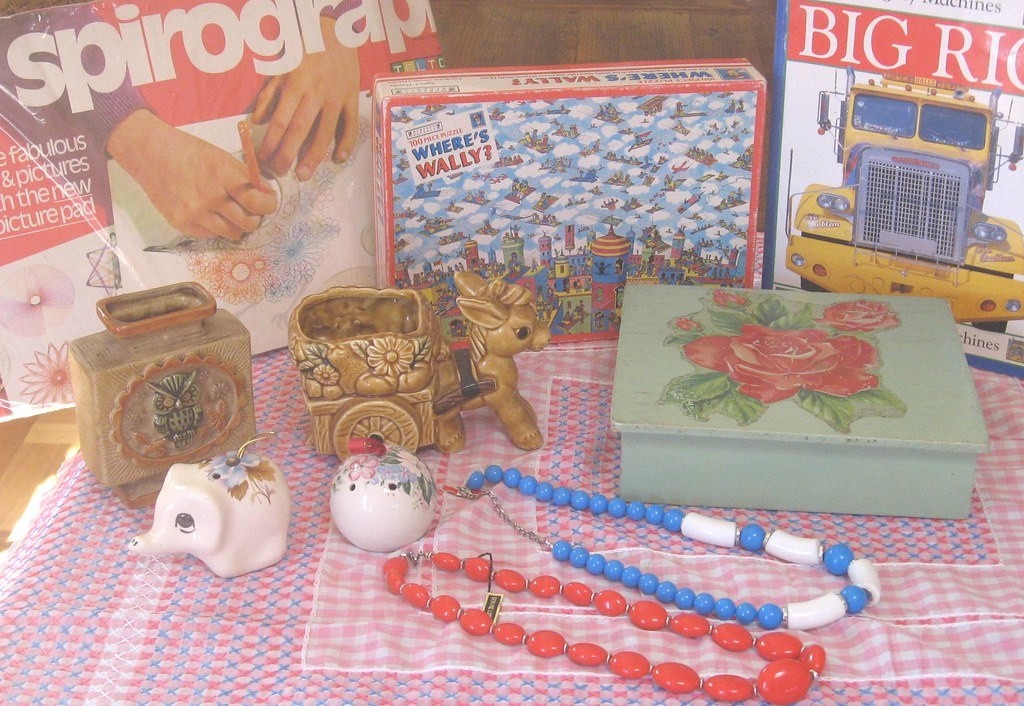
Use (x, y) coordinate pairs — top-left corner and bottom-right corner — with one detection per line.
(0, 344), (1024, 706)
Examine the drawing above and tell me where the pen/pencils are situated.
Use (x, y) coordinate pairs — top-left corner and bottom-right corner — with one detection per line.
(234, 120), (261, 190)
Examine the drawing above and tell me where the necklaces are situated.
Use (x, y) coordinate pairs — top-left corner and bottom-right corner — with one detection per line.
(454, 465), (880, 630)
(384, 551), (825, 706)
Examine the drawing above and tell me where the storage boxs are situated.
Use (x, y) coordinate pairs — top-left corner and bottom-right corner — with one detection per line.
(370, 59), (768, 347)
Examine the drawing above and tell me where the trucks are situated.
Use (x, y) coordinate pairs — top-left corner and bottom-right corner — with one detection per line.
(782, 64), (1024, 333)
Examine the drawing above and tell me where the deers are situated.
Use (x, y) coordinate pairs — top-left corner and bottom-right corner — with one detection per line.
(434, 270), (554, 455)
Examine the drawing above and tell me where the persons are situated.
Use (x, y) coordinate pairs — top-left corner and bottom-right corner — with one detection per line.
(21, 0), (360, 242)
